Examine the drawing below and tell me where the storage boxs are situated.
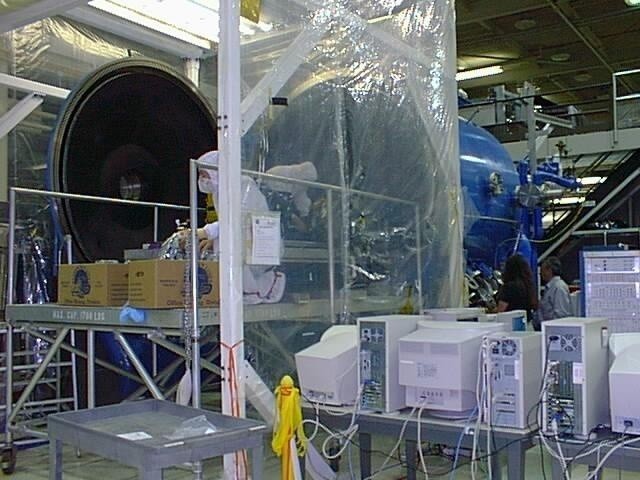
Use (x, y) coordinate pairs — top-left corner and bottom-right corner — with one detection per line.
(57, 259), (220, 309)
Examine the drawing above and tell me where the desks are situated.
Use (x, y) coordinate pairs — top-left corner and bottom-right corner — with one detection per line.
(536, 418), (639, 478)
(48, 399), (269, 478)
(299, 403), (539, 478)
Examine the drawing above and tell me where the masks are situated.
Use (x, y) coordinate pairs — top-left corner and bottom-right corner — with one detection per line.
(198, 176), (217, 193)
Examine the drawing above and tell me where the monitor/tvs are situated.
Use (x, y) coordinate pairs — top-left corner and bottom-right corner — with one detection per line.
(607, 333), (640, 435)
(424, 307), (485, 322)
(398, 321), (513, 420)
(295, 325), (357, 406)
(478, 310), (528, 331)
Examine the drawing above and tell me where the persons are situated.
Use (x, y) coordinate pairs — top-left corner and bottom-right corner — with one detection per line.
(494, 253), (539, 331)
(178, 150), (347, 302)
(532, 255), (574, 331)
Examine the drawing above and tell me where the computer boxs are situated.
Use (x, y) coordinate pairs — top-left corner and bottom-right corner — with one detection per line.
(542, 317), (611, 437)
(357, 315), (430, 412)
(482, 331), (541, 429)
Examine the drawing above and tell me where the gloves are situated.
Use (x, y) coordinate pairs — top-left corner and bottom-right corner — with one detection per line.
(199, 239), (213, 252)
(180, 229), (205, 244)
